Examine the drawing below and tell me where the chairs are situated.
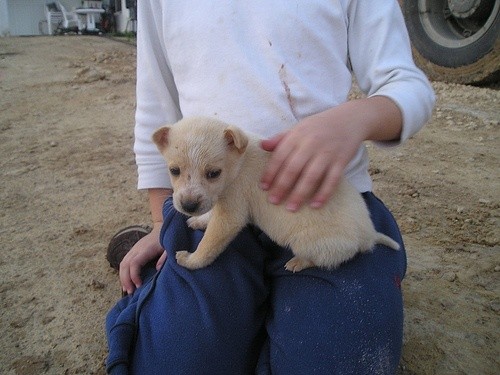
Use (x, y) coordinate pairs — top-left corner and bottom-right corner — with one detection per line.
(57, 2), (82, 35)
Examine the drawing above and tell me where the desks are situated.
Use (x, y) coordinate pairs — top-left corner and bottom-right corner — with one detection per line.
(47, 12), (77, 35)
(75, 8), (105, 30)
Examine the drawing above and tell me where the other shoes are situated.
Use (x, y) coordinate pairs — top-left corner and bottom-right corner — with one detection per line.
(107, 225), (161, 275)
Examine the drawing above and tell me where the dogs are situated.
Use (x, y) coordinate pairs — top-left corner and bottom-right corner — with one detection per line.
(151, 117), (399, 274)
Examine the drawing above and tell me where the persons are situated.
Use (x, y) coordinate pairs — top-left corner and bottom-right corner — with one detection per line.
(105, 0), (436, 375)
